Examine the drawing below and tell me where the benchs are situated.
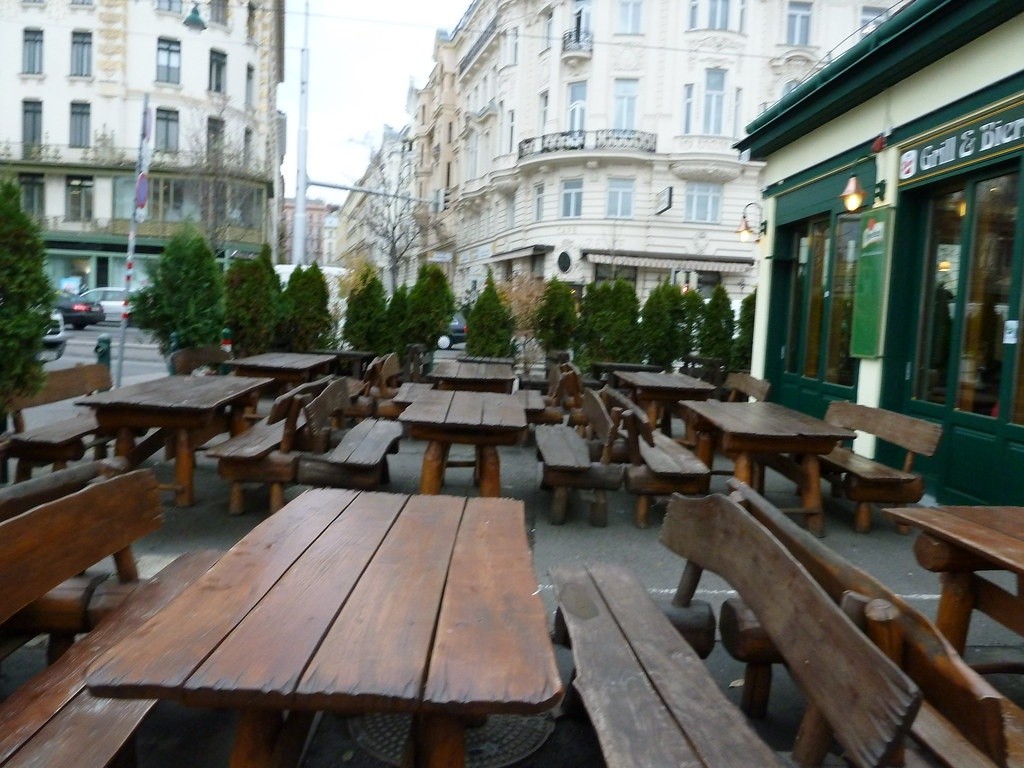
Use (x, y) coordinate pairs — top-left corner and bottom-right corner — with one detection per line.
(1, 341), (1024, 768)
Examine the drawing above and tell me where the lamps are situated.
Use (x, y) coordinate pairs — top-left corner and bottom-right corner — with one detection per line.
(184, 4), (205, 36)
(734, 202), (767, 241)
(840, 173), (886, 211)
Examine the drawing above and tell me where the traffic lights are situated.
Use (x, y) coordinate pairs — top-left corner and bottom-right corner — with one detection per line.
(437, 187), (451, 213)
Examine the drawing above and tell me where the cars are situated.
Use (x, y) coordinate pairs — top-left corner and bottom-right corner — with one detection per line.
(78, 286), (153, 327)
(50, 288), (106, 331)
(29, 304), (67, 365)
(436, 311), (468, 350)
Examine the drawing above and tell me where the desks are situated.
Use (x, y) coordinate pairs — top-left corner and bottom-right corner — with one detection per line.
(87, 486), (562, 768)
(678, 399), (858, 538)
(308, 348), (374, 380)
(878, 507), (1024, 654)
(455, 355), (515, 367)
(224, 352), (337, 425)
(71, 374), (274, 507)
(592, 361), (665, 387)
(397, 389), (530, 496)
(430, 362), (514, 394)
(611, 371), (718, 438)
